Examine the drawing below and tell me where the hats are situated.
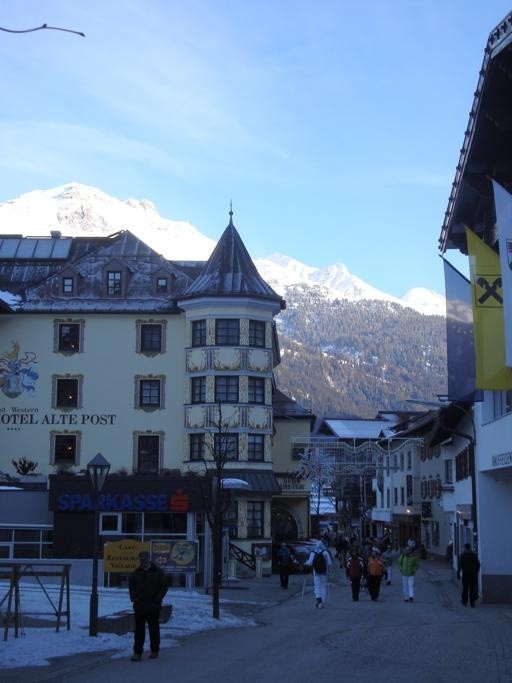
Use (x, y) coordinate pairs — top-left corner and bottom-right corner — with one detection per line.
(137, 551), (150, 560)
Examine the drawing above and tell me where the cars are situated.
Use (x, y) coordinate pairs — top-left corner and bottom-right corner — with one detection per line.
(278, 520), (360, 573)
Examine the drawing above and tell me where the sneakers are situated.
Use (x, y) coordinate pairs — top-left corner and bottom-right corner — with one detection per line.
(149, 652), (158, 658)
(131, 654), (140, 661)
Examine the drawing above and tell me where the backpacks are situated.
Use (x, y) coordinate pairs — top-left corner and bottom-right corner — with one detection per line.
(310, 550), (327, 574)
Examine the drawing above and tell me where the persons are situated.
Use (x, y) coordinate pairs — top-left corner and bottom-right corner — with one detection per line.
(456, 543), (480, 608)
(323, 529), (428, 602)
(128, 551), (168, 662)
(276, 543), (293, 590)
(304, 540), (333, 609)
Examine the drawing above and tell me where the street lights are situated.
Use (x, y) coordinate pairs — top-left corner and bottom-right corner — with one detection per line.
(406, 398), (476, 439)
(85, 452), (110, 635)
